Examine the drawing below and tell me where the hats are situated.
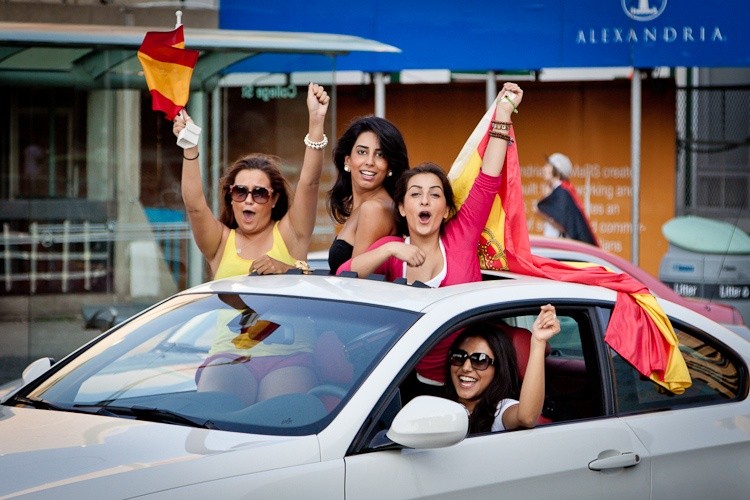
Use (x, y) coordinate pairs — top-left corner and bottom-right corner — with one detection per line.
(547, 153), (572, 178)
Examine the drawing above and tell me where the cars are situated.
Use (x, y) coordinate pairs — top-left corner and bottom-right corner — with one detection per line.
(1, 266), (749, 500)
(304, 235), (743, 361)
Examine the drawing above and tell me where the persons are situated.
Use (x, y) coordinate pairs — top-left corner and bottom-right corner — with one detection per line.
(248, 118), (410, 383)
(335, 81), (523, 394)
(442, 303), (561, 435)
(537, 153), (600, 247)
(172, 82), (329, 404)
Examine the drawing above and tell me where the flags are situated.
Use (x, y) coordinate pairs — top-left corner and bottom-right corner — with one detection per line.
(443, 91), (692, 395)
(137, 24), (200, 120)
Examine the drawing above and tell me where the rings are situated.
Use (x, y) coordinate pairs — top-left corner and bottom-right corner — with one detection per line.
(185, 117), (190, 120)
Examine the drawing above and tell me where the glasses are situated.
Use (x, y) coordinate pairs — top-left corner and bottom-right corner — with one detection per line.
(449, 348), (495, 371)
(228, 185), (275, 204)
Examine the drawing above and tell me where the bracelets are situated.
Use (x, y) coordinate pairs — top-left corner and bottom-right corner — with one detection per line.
(489, 131), (510, 142)
(183, 152), (199, 160)
(491, 121), (512, 129)
(304, 133), (329, 150)
(296, 260), (308, 274)
